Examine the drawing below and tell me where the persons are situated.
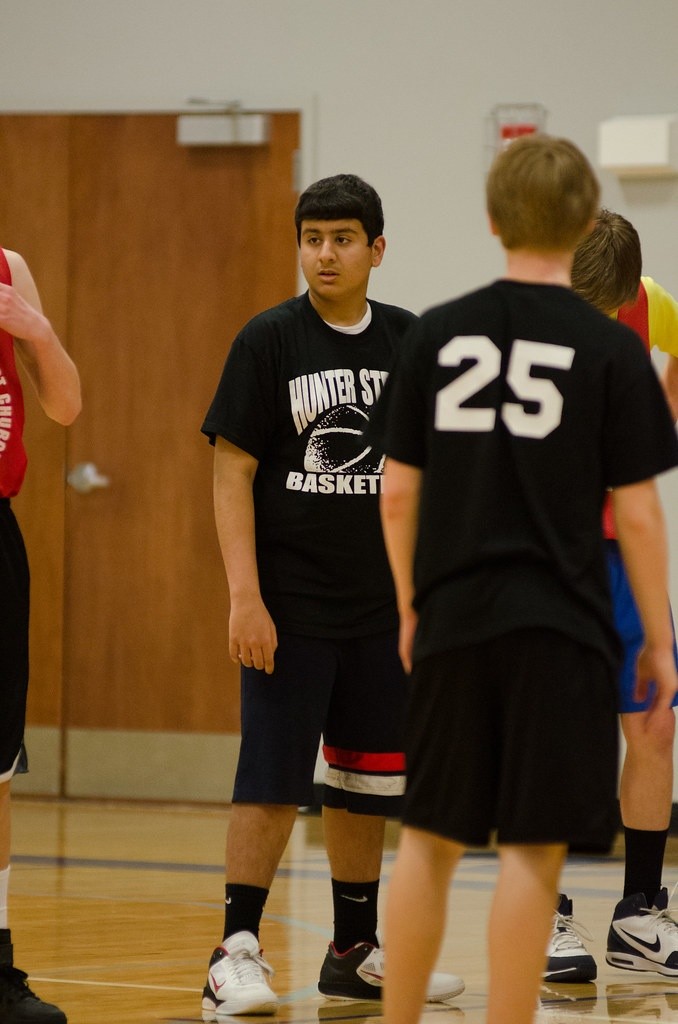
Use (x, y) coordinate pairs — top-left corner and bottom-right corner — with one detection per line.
(201, 133), (678, 1024)
(0, 243), (83, 1024)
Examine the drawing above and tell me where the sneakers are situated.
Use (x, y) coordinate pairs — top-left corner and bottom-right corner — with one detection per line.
(0, 929), (67, 1024)
(317, 934), (464, 1002)
(201, 930), (279, 1015)
(605, 887), (678, 977)
(542, 893), (597, 981)
